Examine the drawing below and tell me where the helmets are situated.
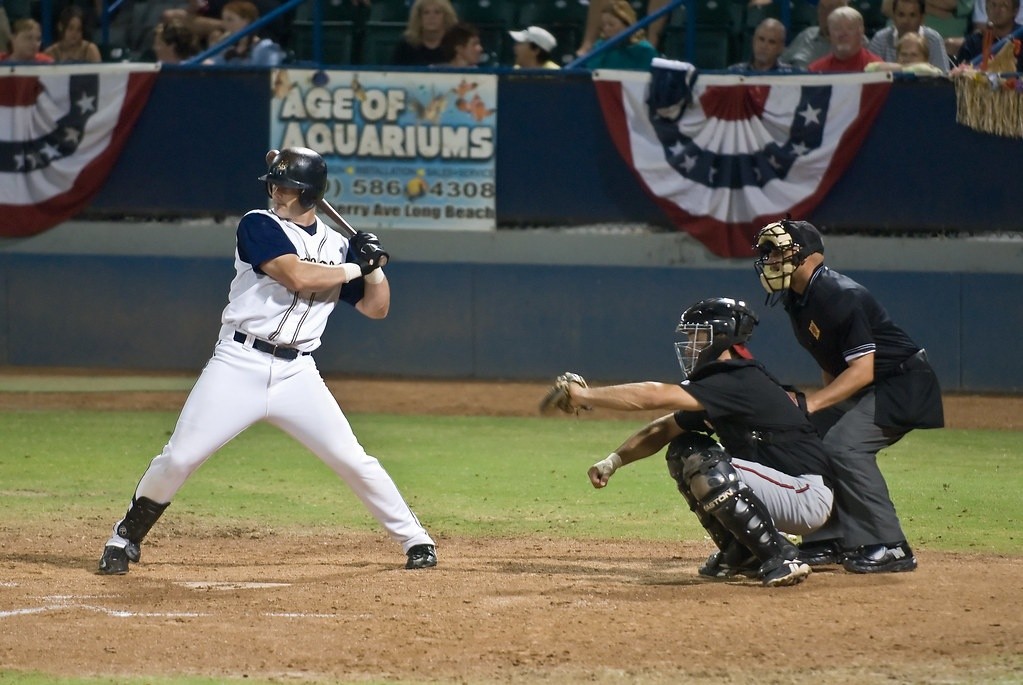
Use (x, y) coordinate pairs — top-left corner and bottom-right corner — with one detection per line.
(258, 146), (328, 209)
(674, 297), (760, 382)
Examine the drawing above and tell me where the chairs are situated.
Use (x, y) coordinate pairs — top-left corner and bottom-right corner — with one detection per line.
(0, 0), (886, 69)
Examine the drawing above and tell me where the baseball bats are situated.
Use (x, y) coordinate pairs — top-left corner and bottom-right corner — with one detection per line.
(265, 148), (389, 267)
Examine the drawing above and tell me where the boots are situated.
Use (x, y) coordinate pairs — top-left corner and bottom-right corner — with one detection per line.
(679, 485), (763, 576)
(710, 487), (812, 586)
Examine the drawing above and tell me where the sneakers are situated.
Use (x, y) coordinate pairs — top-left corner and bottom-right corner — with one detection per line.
(406, 544), (438, 568)
(843, 541), (917, 573)
(797, 538), (843, 566)
(98, 540), (129, 575)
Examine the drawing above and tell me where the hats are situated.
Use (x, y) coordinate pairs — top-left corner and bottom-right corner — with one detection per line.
(508, 26), (558, 53)
(759, 220), (824, 261)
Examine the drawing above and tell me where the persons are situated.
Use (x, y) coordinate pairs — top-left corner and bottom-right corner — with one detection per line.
(752, 215), (945, 574)
(98, 148), (438, 575)
(0, 0), (288, 66)
(726, 0), (1023, 76)
(538, 297), (834, 589)
(393, 0), (668, 70)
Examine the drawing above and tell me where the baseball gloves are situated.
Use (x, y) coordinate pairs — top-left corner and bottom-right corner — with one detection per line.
(537, 371), (595, 419)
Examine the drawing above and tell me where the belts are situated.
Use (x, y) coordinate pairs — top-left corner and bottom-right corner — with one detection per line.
(233, 330), (311, 360)
(885, 348), (929, 380)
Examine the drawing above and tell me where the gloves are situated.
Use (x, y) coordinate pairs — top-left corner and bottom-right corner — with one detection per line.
(349, 243), (390, 278)
(349, 229), (381, 260)
